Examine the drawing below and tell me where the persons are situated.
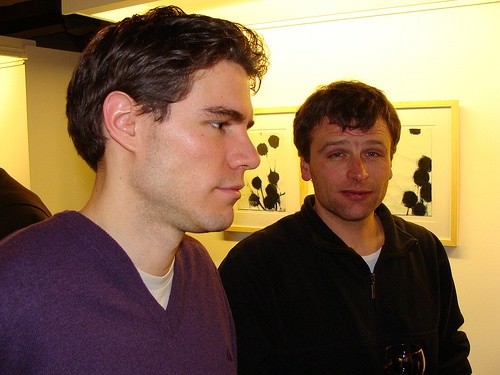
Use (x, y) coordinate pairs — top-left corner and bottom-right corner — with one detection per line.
(0, 6), (268, 375)
(0, 167), (52, 240)
(218, 81), (472, 375)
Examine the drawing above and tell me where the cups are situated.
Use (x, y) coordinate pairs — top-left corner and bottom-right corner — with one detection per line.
(381, 343), (426, 375)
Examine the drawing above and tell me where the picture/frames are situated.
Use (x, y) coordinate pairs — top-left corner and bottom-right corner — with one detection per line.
(382, 100), (460, 247)
(223, 105), (308, 233)
